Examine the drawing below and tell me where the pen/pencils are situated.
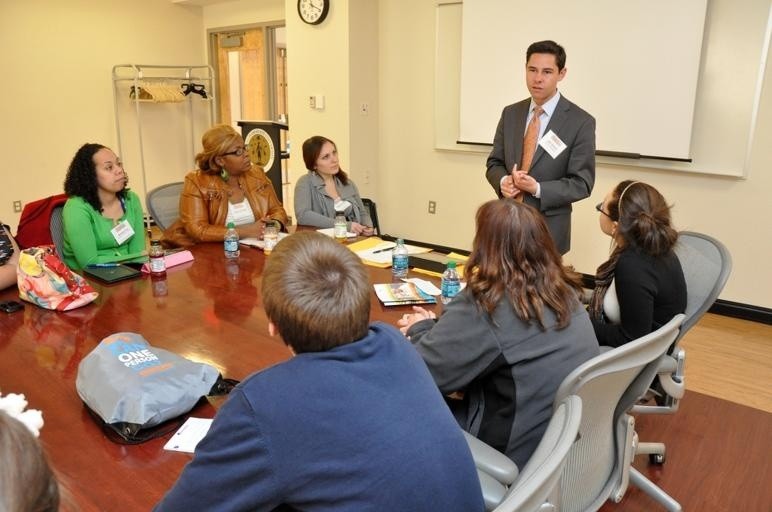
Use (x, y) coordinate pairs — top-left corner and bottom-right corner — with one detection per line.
(87, 263), (121, 267)
(373, 247), (394, 253)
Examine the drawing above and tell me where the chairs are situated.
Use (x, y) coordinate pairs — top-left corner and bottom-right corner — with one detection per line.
(595, 230), (735, 461)
(536, 311), (687, 511)
(455, 394), (582, 512)
(360, 198), (381, 238)
(146, 182), (184, 232)
(49, 204), (71, 262)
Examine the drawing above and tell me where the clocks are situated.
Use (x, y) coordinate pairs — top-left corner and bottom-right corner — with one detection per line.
(296, 0), (330, 26)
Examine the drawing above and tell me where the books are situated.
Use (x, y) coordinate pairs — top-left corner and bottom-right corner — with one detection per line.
(384, 300), (436, 306)
(84, 263), (140, 284)
(238, 231), (291, 250)
(373, 281), (435, 303)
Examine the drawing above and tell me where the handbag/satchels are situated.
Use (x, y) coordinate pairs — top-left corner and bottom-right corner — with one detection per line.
(16, 245), (100, 311)
(76, 332), (220, 443)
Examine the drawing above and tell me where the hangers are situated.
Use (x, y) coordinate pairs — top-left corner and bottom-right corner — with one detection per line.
(127, 78), (215, 104)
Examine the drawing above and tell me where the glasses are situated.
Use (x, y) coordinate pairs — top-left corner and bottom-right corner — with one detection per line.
(596, 203), (617, 221)
(219, 144), (248, 156)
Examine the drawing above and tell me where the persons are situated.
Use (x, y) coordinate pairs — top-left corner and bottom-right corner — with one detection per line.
(159, 124), (289, 248)
(561, 181), (688, 403)
(1, 220), (20, 292)
(484, 40), (596, 258)
(294, 136), (376, 238)
(396, 199), (601, 487)
(142, 231), (489, 512)
(179, 239), (268, 324)
(60, 142), (149, 280)
(83, 276), (147, 345)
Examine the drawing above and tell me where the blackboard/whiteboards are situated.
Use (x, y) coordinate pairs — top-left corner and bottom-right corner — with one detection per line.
(434, 0), (772, 180)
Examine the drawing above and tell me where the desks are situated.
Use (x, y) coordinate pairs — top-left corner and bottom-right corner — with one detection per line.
(2, 219), (472, 511)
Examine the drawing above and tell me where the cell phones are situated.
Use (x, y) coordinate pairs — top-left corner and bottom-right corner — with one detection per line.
(1, 299), (25, 313)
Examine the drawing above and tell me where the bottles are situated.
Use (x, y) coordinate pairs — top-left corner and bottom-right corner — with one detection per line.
(262, 220), (278, 255)
(147, 238), (166, 277)
(441, 260), (461, 306)
(223, 259), (241, 291)
(333, 210), (348, 244)
(391, 237), (409, 277)
(222, 221), (240, 259)
(150, 278), (169, 311)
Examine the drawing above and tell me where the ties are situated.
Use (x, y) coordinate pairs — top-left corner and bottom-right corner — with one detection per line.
(515, 107), (545, 206)
